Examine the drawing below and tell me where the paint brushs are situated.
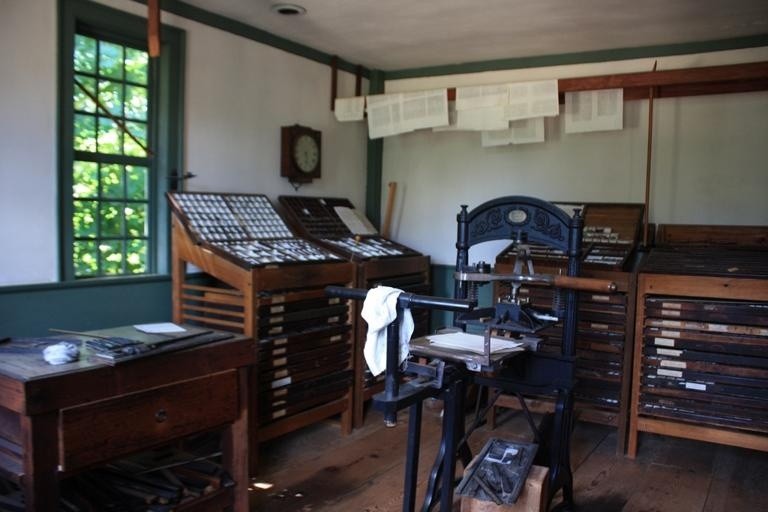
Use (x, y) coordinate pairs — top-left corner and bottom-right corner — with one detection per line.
(148, 331), (213, 349)
(49, 328), (112, 340)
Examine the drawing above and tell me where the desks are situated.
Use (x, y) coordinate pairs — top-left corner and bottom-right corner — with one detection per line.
(0, 321), (259, 511)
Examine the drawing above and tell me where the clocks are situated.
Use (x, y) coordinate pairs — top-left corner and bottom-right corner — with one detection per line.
(280, 121), (323, 185)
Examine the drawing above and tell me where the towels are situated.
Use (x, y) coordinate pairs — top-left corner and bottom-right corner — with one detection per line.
(361, 285), (415, 379)
